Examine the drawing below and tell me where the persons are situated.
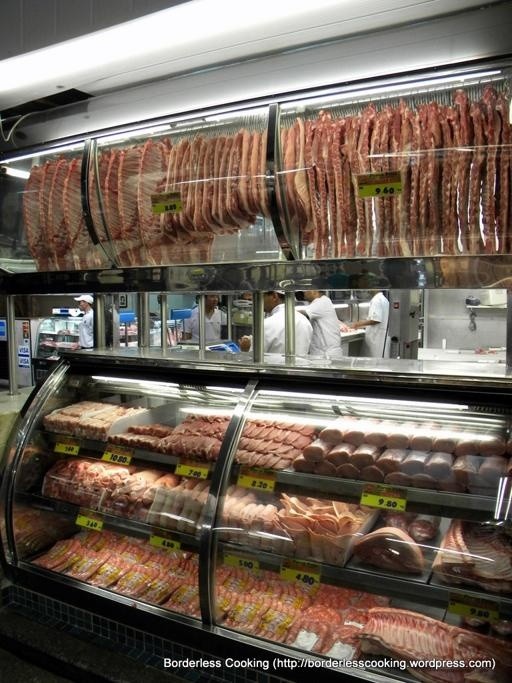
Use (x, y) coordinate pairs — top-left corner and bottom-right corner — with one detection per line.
(192, 295), (200, 309)
(237, 289), (313, 355)
(23, 322), (29, 339)
(296, 290), (343, 356)
(182, 295), (228, 341)
(242, 291), (252, 300)
(352, 288), (392, 358)
(71, 294), (94, 351)
(120, 296), (125, 306)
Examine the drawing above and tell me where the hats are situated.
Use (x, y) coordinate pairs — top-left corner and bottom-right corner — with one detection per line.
(74, 295), (94, 304)
